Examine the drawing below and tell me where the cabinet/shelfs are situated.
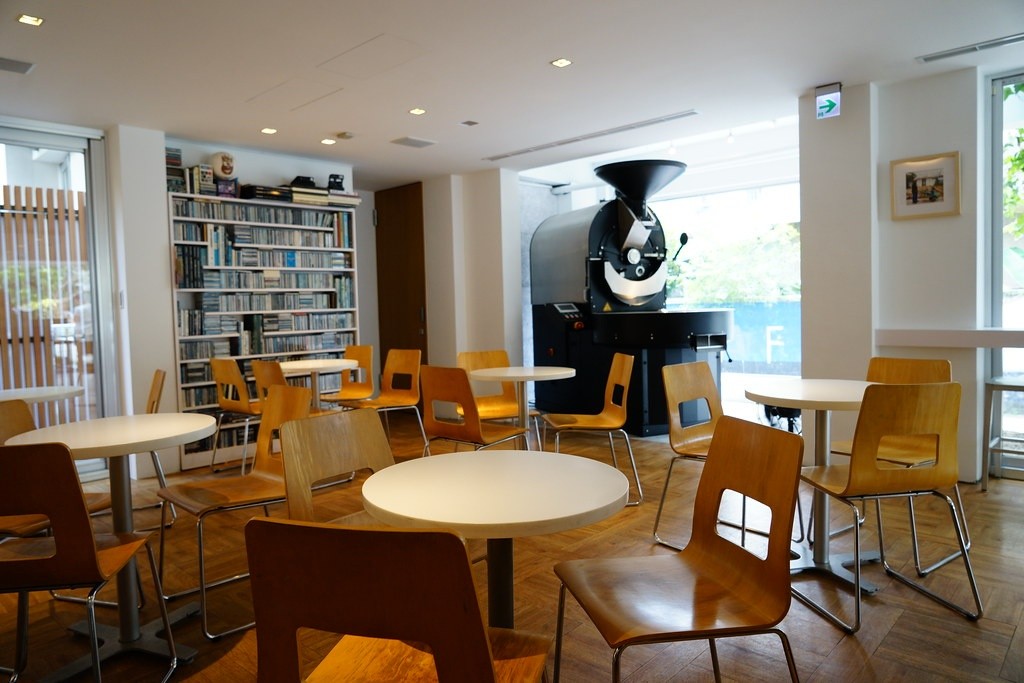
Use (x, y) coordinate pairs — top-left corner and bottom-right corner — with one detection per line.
(167, 191), (363, 473)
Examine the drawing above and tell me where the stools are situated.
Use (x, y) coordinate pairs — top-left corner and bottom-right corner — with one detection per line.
(983, 376), (1024, 491)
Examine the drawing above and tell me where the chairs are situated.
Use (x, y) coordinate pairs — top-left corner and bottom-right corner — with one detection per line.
(789, 383), (985, 635)
(339, 349), (433, 456)
(210, 358), (268, 474)
(806, 357), (972, 578)
(252, 360), (356, 492)
(322, 344), (375, 413)
(422, 364), (532, 458)
(553, 413), (806, 683)
(653, 361), (805, 554)
(454, 350), (544, 451)
(1, 369), (314, 682)
(245, 516), (552, 683)
(542, 353), (644, 507)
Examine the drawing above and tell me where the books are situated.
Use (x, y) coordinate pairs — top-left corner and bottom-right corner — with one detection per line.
(172, 246), (355, 289)
(172, 197), (349, 248)
(165, 147), (362, 208)
(183, 392), (362, 462)
(178, 353), (356, 408)
(175, 290), (356, 360)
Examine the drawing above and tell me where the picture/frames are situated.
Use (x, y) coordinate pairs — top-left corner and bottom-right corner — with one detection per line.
(889, 149), (962, 221)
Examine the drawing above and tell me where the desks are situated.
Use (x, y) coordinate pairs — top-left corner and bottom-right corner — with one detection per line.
(745, 379), (884, 596)
(280, 359), (360, 411)
(0, 411), (217, 682)
(472, 367), (577, 448)
(1, 384), (85, 405)
(360, 451), (633, 631)
